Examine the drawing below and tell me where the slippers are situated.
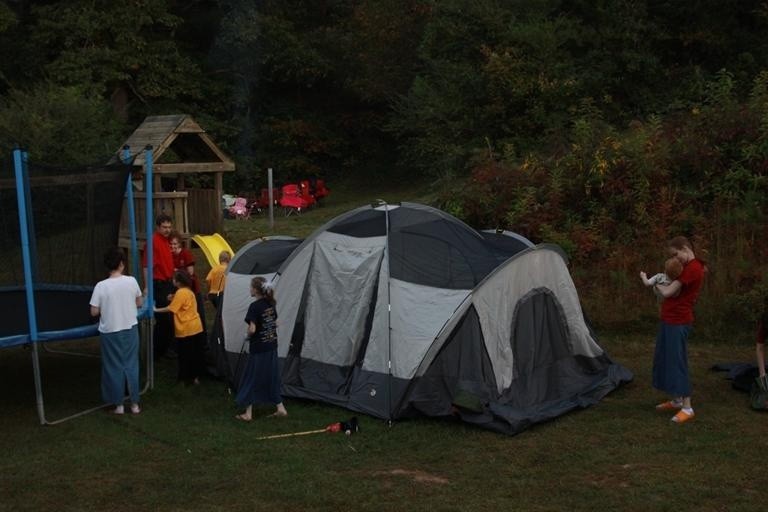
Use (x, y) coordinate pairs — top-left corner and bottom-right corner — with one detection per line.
(235, 413), (251, 423)
(655, 400), (681, 411)
(672, 409), (695, 423)
(266, 413), (288, 418)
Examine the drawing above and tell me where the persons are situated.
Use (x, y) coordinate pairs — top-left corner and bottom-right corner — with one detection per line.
(204, 250), (232, 310)
(652, 235), (705, 423)
(141, 214), (177, 358)
(153, 271), (205, 398)
(754, 294), (768, 377)
(169, 232), (208, 333)
(235, 274), (288, 422)
(86, 248), (143, 413)
(640, 257), (684, 304)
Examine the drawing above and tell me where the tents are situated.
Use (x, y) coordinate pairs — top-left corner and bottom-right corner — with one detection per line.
(210, 201), (634, 438)
